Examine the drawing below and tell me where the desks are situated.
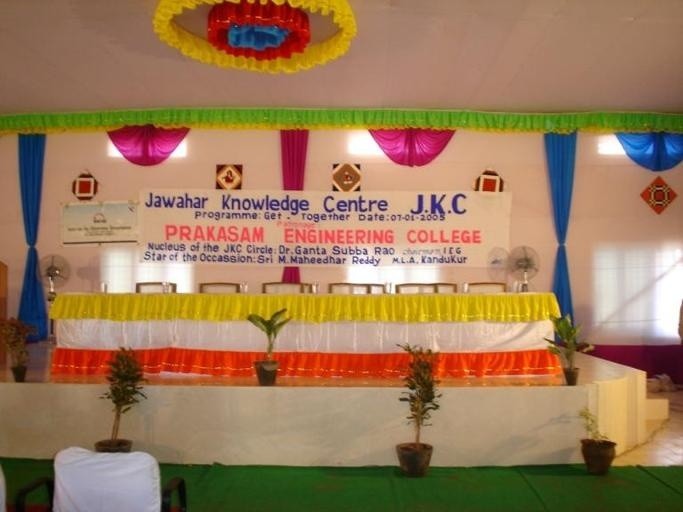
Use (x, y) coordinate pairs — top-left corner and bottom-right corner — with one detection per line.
(53, 293), (563, 378)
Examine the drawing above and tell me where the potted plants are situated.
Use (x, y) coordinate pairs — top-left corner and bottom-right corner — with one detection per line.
(395, 343), (443, 476)
(579, 409), (617, 474)
(542, 314), (594, 385)
(0, 316), (40, 382)
(247, 308), (292, 387)
(94, 346), (148, 452)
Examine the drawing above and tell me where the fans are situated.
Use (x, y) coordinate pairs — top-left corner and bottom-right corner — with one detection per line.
(507, 245), (540, 293)
(35, 254), (71, 346)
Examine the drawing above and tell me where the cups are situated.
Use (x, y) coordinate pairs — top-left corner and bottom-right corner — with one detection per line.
(239, 280), (248, 292)
(460, 281), (469, 294)
(98, 280), (108, 293)
(511, 280), (522, 292)
(383, 282), (391, 294)
(311, 280), (320, 294)
(161, 281), (169, 294)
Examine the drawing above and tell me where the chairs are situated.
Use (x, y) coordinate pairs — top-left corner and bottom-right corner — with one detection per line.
(54, 445), (187, 512)
(0, 462), (53, 512)
(199, 282), (240, 293)
(262, 281), (304, 293)
(465, 282), (507, 292)
(136, 282), (176, 292)
(329, 283), (371, 294)
(369, 283), (385, 294)
(437, 283), (457, 293)
(396, 282), (439, 293)
(302, 283), (317, 294)
